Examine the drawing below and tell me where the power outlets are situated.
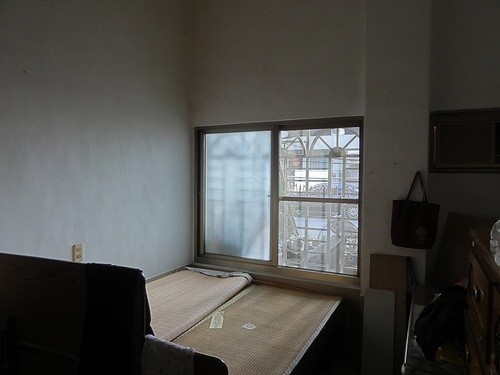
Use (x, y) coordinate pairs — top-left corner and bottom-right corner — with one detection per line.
(73, 242), (83, 263)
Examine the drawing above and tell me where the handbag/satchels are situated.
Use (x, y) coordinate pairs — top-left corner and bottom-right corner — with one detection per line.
(391, 171), (440, 249)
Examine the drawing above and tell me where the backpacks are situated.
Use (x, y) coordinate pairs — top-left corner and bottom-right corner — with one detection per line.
(414, 285), (469, 361)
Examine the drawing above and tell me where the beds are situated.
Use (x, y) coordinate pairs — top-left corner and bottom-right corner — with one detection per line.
(1, 253), (344, 375)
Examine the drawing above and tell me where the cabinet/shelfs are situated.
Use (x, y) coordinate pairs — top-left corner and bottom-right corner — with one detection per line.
(457, 228), (500, 375)
